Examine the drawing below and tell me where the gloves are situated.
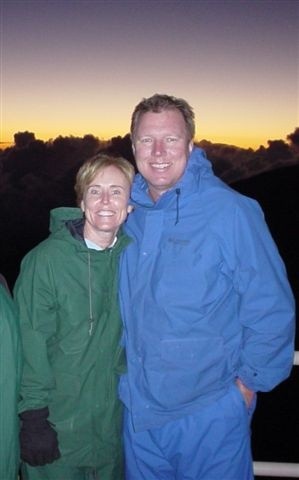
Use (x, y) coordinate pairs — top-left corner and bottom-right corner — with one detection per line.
(19, 406), (61, 467)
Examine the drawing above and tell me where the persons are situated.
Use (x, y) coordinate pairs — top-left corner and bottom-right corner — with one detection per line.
(116, 93), (298, 480)
(1, 273), (21, 480)
(13, 153), (127, 480)
